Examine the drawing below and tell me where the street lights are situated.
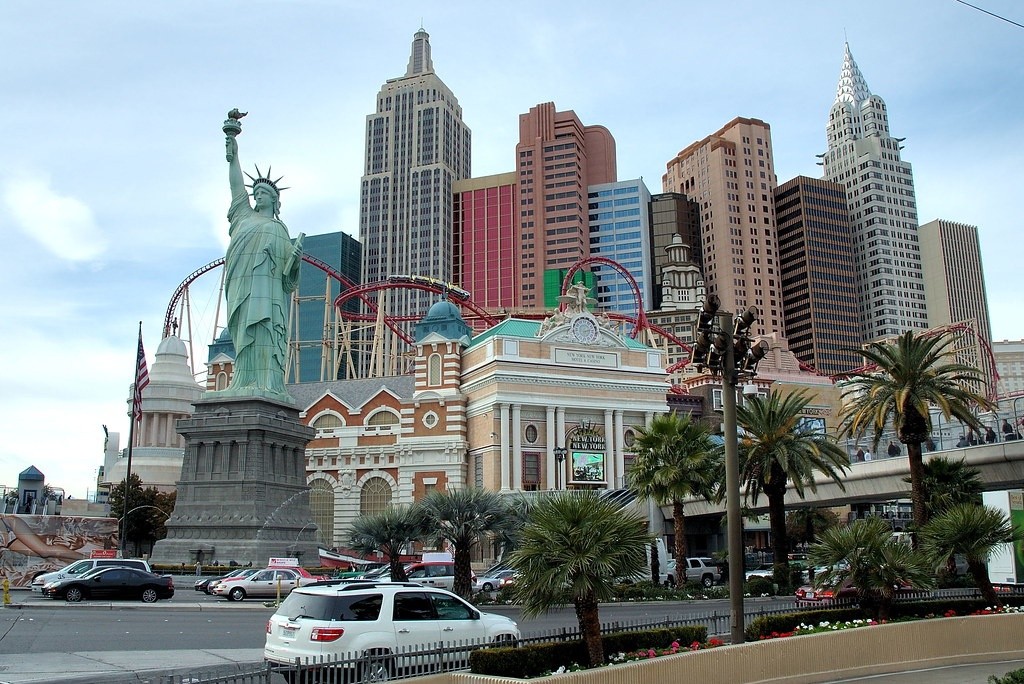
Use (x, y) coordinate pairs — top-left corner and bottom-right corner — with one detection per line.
(689, 293), (770, 646)
(552, 446), (568, 490)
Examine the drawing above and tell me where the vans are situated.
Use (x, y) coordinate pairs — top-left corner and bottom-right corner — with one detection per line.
(30, 558), (151, 595)
(602, 535), (669, 597)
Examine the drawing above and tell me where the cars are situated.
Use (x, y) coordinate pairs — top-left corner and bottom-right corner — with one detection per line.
(47, 566), (175, 603)
(668, 557), (721, 588)
(193, 557), (573, 605)
(41, 566), (139, 600)
(745, 557), (904, 607)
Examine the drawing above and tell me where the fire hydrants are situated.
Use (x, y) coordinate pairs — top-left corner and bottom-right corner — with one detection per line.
(0, 577), (13, 604)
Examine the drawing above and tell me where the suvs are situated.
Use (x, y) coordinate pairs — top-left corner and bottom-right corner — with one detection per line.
(262, 578), (524, 684)
